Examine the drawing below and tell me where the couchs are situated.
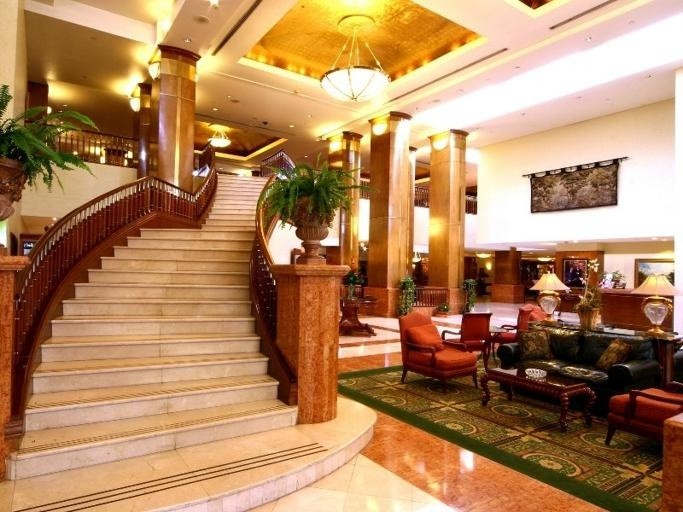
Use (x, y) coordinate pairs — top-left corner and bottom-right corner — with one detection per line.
(497, 324), (661, 418)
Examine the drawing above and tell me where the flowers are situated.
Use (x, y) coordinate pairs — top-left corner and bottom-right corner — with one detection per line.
(612, 270), (625, 280)
(436, 302), (448, 311)
(341, 268), (368, 286)
(573, 258), (601, 309)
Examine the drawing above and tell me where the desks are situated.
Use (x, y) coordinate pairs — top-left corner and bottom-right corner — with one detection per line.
(479, 368), (596, 432)
(489, 326), (507, 365)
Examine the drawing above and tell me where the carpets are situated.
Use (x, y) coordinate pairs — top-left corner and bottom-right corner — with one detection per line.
(334, 352), (663, 512)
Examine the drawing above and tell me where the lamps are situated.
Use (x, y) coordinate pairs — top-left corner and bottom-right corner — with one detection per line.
(476, 252), (491, 259)
(537, 257), (552, 261)
(630, 275), (681, 334)
(207, 124), (231, 148)
(318, 15), (391, 108)
(528, 273), (570, 321)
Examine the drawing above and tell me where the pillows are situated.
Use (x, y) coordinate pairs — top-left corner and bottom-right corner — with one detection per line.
(594, 338), (633, 371)
(517, 329), (555, 360)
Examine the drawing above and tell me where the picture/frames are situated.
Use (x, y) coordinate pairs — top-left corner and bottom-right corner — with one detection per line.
(633, 258), (674, 288)
(561, 258), (589, 288)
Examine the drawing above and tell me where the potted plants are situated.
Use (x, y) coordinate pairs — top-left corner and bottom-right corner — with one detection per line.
(394, 274), (416, 318)
(258, 150), (376, 264)
(463, 278), (477, 312)
(0, 84), (100, 222)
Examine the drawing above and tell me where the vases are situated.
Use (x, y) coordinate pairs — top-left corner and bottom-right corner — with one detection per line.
(434, 309), (448, 317)
(576, 308), (599, 331)
(346, 284), (354, 301)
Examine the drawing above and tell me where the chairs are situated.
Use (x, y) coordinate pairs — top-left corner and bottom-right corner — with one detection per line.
(500, 308), (533, 331)
(399, 311), (478, 395)
(441, 313), (493, 371)
(604, 381), (683, 445)
